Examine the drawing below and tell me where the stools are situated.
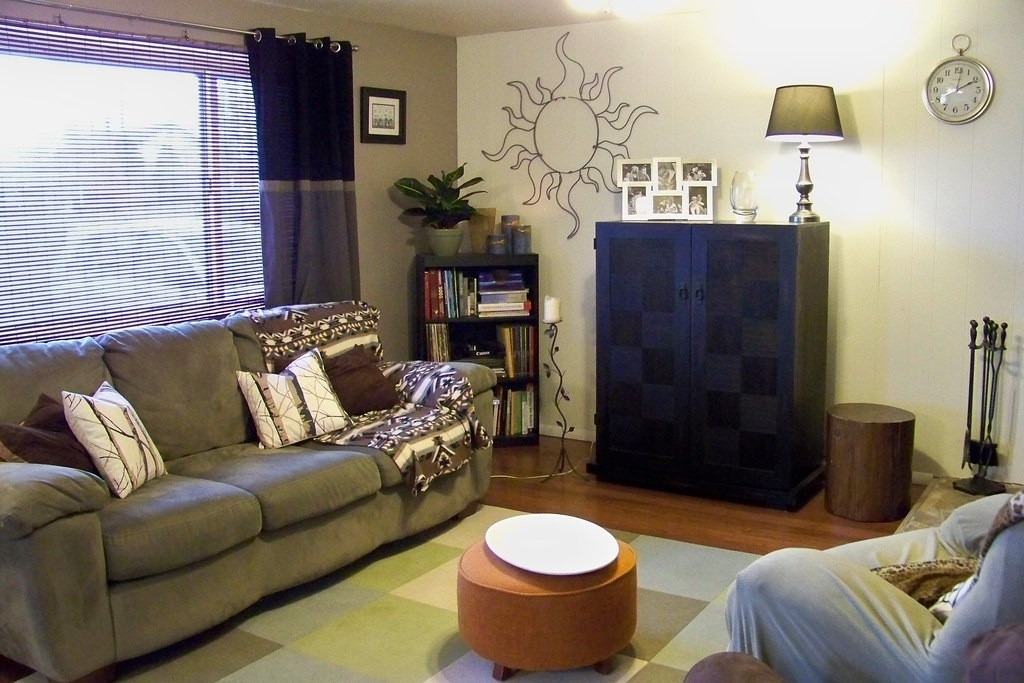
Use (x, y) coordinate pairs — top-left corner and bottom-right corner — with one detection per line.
(457, 537), (639, 681)
(824, 404), (916, 523)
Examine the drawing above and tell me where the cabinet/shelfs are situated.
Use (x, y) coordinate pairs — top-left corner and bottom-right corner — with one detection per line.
(414, 252), (540, 448)
(593, 219), (830, 513)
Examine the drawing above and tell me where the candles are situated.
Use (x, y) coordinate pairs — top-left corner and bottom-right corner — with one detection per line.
(542, 294), (563, 323)
(500, 215), (520, 253)
(486, 233), (510, 255)
(511, 224), (532, 254)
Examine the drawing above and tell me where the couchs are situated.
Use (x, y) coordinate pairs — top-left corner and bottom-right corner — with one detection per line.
(723, 492), (1024, 683)
(0, 299), (497, 683)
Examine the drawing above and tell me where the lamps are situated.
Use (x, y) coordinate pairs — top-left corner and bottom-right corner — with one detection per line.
(765, 83), (845, 223)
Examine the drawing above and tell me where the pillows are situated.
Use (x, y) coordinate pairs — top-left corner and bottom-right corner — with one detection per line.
(0, 392), (100, 476)
(274, 344), (400, 415)
(236, 347), (357, 450)
(60, 381), (169, 500)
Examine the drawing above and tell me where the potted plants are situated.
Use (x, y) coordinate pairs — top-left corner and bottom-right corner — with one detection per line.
(393, 161), (488, 256)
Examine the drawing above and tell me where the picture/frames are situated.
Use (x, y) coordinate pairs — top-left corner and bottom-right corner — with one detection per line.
(682, 184), (714, 221)
(621, 181), (651, 221)
(616, 158), (654, 187)
(681, 159), (718, 187)
(359, 85), (407, 145)
(651, 156), (683, 193)
(648, 191), (689, 220)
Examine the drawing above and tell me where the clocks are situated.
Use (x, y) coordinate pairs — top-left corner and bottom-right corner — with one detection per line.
(923, 34), (996, 125)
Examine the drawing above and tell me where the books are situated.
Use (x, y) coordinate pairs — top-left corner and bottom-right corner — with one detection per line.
(427, 323), (536, 380)
(424, 266), (532, 316)
(488, 384), (535, 439)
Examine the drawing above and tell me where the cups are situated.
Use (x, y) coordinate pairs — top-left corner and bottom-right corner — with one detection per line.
(511, 225), (533, 253)
(486, 234), (508, 253)
(469, 206), (496, 253)
(728, 171), (759, 217)
(501, 215), (520, 253)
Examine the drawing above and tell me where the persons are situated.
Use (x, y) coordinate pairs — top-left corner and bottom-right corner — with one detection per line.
(374, 109), (393, 128)
(623, 162), (707, 215)
